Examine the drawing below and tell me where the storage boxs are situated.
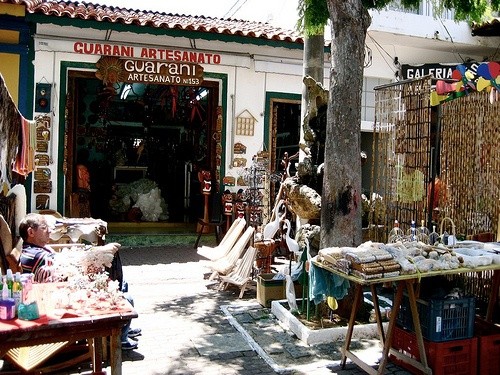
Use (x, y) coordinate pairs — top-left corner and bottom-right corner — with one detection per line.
(452, 247), (493, 266)
(256, 273), (308, 308)
(389, 326), (479, 375)
(395, 290), (476, 343)
(475, 317), (500, 375)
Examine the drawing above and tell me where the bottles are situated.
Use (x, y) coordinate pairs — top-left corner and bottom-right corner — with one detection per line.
(6, 275), (14, 298)
(389, 218), (440, 245)
(12, 272), (23, 304)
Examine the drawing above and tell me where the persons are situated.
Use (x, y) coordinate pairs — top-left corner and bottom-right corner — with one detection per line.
(19, 213), (142, 350)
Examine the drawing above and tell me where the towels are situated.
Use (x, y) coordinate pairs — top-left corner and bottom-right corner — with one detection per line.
(29, 119), (37, 151)
(12, 116), (34, 180)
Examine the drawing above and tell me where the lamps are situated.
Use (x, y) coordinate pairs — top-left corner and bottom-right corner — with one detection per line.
(188, 88), (209, 104)
(119, 82), (133, 100)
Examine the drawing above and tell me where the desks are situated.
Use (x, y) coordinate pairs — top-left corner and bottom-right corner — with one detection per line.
(113, 165), (148, 184)
(310, 259), (500, 375)
(0, 281), (139, 375)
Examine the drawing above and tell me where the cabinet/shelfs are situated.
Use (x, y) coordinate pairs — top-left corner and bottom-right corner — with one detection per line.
(246, 167), (274, 273)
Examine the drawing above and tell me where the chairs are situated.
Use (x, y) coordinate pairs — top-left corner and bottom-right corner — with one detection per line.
(6, 237), (85, 275)
(196, 217), (246, 272)
(203, 217), (242, 250)
(198, 226), (256, 289)
(218, 245), (257, 300)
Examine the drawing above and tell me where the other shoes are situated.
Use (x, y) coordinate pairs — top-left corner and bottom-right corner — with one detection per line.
(107, 337), (138, 349)
(128, 327), (141, 336)
